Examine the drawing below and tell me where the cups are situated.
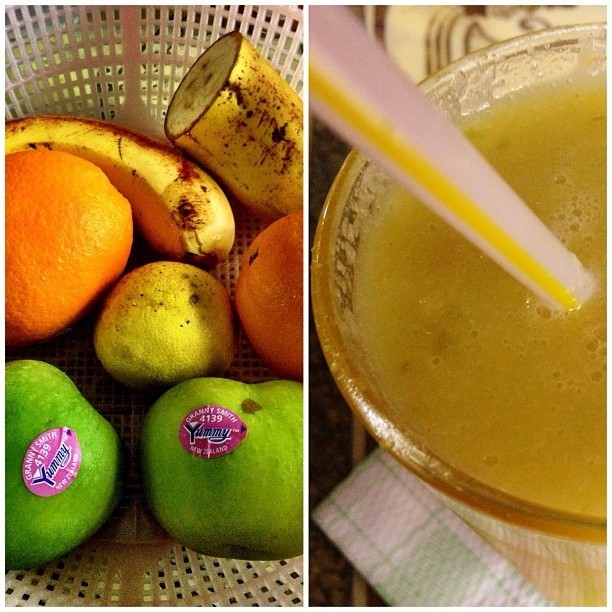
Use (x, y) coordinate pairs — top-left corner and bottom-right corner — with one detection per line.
(316, 15), (611, 560)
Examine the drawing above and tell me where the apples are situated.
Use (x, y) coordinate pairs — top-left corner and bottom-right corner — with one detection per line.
(141, 378), (304, 562)
(6, 359), (124, 567)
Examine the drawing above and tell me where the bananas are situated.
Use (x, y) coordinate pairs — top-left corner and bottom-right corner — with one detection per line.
(6, 116), (237, 272)
(164, 30), (302, 224)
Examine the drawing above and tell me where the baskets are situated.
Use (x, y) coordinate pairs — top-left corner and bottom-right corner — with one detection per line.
(7, 8), (305, 608)
